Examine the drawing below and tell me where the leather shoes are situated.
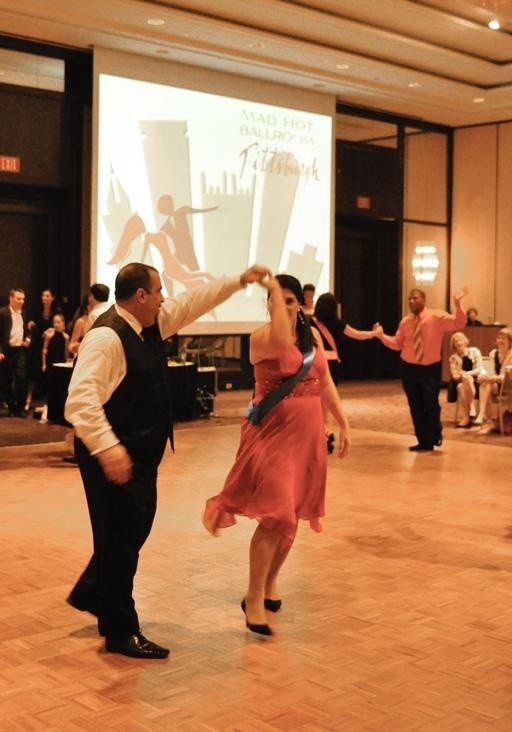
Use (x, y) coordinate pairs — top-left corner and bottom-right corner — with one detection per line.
(468, 408), (477, 418)
(104, 629), (170, 659)
(263, 598), (282, 613)
(63, 455), (78, 464)
(409, 444), (434, 451)
(9, 409), (28, 419)
(476, 423), (497, 436)
(97, 617), (107, 637)
(240, 597), (273, 637)
(433, 433), (442, 446)
(326, 432), (335, 454)
(452, 420), (473, 428)
(474, 413), (487, 425)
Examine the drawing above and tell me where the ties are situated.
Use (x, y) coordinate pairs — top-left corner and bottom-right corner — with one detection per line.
(411, 315), (425, 364)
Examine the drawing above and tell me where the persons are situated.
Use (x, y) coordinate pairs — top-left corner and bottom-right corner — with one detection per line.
(306, 292), (385, 454)
(370, 284), (469, 455)
(234, 267), (353, 636)
(446, 330), (489, 429)
(0, 283), (113, 430)
(465, 306), (484, 325)
(301, 285), (316, 319)
(477, 328), (511, 437)
(63, 262), (271, 660)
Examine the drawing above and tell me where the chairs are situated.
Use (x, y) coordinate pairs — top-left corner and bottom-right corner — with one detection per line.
(489, 352), (512, 434)
(454, 354), (490, 428)
